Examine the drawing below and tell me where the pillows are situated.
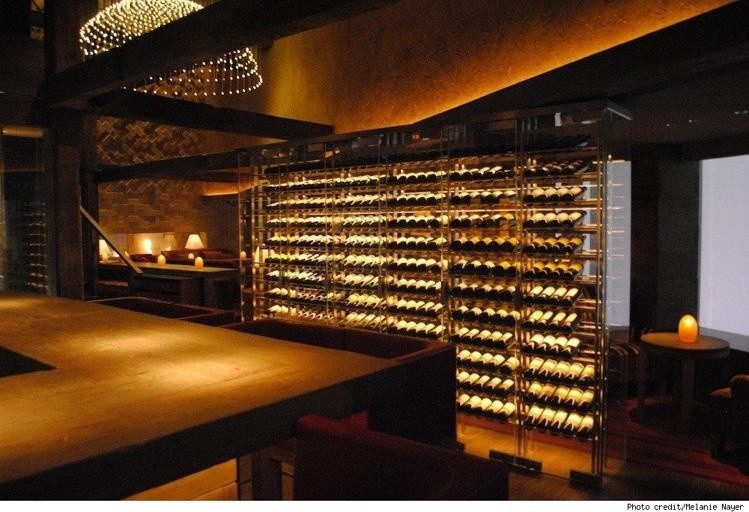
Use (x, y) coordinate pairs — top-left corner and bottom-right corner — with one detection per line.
(177, 250), (223, 261)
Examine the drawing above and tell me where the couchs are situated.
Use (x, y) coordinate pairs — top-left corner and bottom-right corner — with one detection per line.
(161, 248), (234, 268)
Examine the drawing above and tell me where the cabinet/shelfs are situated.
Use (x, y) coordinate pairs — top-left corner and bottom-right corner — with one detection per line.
(455, 341), (596, 443)
(239, 100), (630, 445)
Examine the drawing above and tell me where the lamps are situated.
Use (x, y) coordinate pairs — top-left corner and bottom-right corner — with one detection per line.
(185, 234), (205, 260)
(679, 315), (699, 344)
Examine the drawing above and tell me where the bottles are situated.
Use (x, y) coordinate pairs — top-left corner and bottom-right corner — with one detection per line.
(455, 341), (596, 443)
(236, 157), (596, 342)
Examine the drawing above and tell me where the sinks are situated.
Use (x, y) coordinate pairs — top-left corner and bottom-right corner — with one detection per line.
(161, 248), (234, 268)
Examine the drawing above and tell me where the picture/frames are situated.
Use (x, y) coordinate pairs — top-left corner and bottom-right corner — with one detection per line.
(99, 260), (238, 308)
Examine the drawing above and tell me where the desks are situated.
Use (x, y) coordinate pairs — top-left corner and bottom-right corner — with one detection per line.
(99, 260), (238, 308)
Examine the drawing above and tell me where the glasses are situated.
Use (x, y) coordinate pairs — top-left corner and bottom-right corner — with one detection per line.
(679, 315), (699, 344)
(185, 234), (205, 260)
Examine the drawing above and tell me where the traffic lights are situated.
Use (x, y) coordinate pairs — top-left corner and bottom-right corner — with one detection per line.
(177, 250), (223, 261)
